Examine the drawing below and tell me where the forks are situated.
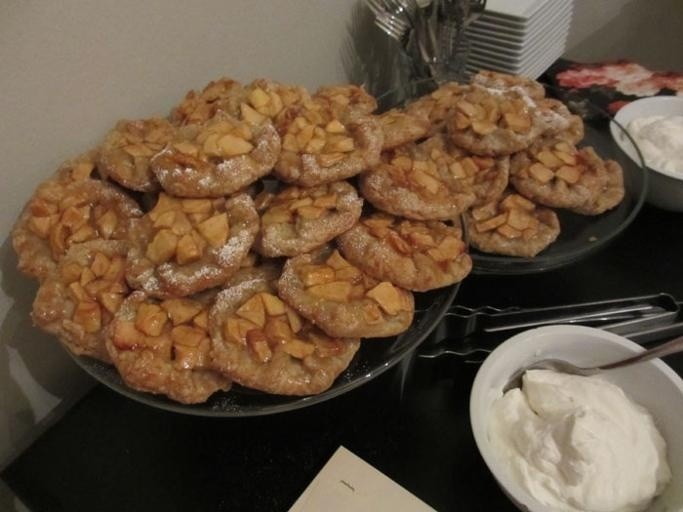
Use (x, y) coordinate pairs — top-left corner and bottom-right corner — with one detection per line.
(363, 0), (488, 56)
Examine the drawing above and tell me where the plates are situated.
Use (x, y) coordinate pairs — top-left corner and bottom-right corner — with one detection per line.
(362, 76), (646, 270)
(63, 277), (462, 416)
(457, 0), (575, 81)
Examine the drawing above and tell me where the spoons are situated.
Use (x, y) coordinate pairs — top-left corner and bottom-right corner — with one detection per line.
(500, 335), (683, 396)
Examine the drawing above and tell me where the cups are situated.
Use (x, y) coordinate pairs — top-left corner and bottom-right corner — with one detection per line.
(398, 34), (470, 106)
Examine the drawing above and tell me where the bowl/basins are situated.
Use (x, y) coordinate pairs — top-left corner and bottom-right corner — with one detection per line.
(468, 323), (683, 512)
(610, 93), (683, 208)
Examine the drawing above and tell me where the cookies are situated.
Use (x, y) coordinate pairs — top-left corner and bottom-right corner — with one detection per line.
(11, 69), (625, 405)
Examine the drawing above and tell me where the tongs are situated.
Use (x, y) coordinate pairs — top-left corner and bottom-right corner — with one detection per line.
(396, 292), (683, 377)
